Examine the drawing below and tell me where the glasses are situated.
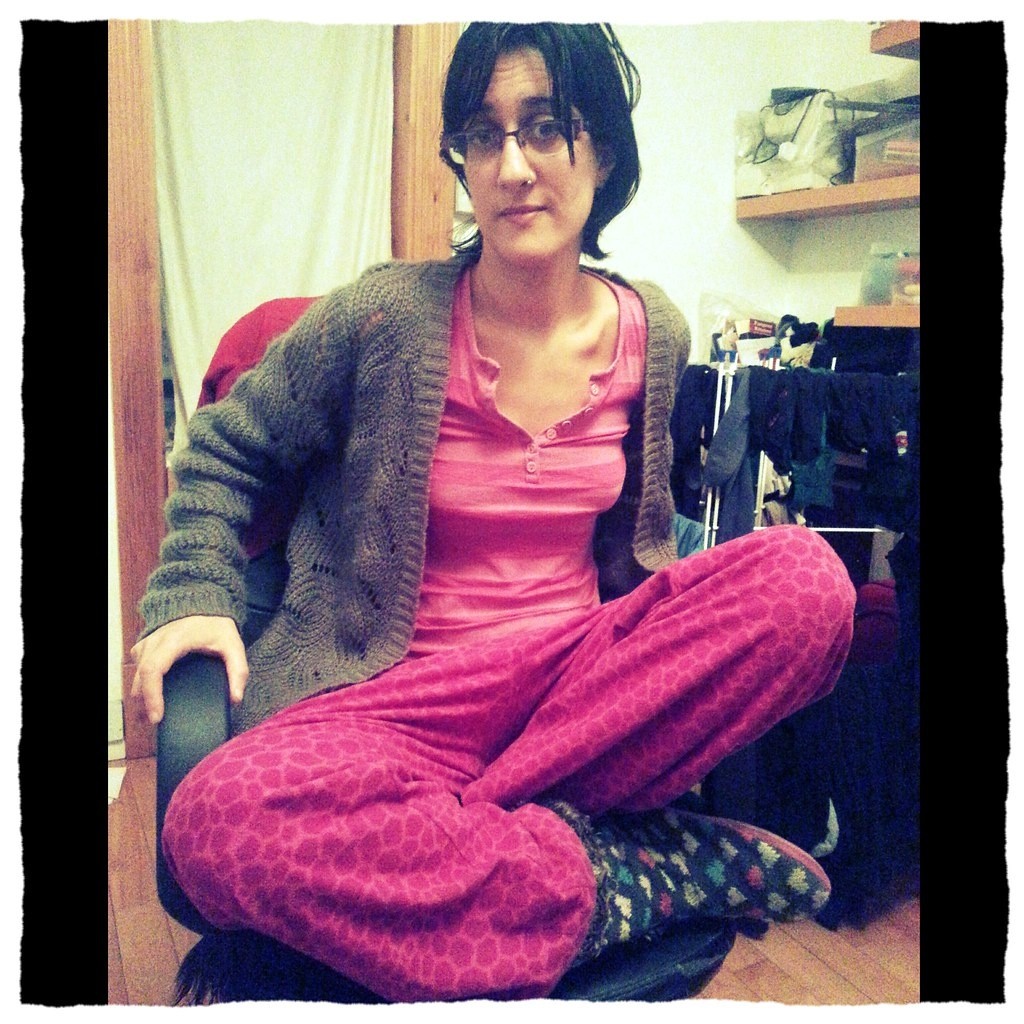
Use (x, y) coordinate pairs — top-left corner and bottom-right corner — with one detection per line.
(439, 119), (588, 159)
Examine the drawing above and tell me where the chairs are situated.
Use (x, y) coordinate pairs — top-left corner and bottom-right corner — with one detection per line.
(156, 294), (734, 1000)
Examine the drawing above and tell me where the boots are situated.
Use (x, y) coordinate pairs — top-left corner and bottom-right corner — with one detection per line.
(548, 801), (833, 964)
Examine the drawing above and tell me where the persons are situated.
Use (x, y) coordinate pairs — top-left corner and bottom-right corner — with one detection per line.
(125, 21), (864, 1001)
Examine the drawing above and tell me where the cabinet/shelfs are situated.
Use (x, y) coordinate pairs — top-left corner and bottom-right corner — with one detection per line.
(734, 20), (920, 330)
